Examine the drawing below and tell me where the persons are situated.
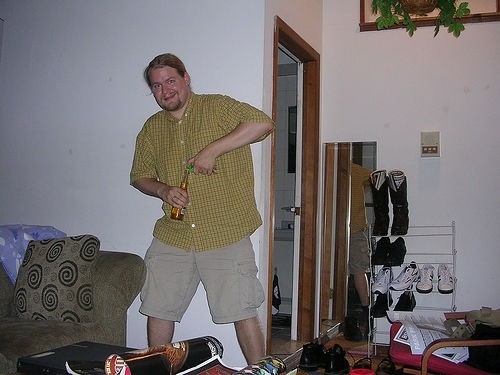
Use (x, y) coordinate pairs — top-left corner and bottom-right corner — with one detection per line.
(129, 53), (276, 366)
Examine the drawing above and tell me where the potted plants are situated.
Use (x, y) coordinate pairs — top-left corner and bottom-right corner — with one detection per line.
(372, 0), (471, 37)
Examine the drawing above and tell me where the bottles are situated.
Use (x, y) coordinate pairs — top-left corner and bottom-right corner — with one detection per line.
(170, 170), (189, 221)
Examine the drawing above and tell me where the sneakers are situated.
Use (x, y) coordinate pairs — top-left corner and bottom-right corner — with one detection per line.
(416, 264), (435, 293)
(438, 264), (453, 294)
(389, 261), (418, 291)
(372, 266), (393, 294)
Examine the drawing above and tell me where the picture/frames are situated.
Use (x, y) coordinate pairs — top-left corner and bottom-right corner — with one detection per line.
(360, 0), (500, 32)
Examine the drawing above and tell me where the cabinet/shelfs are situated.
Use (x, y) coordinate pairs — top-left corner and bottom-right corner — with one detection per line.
(368, 221), (458, 346)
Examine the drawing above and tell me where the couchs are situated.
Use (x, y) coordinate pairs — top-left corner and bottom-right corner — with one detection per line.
(0, 251), (147, 375)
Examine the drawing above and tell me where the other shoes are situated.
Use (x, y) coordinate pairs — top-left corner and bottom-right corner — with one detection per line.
(299, 342), (326, 371)
(370, 291), (393, 318)
(392, 291), (416, 312)
(325, 344), (350, 375)
(385, 237), (406, 265)
(372, 237), (390, 265)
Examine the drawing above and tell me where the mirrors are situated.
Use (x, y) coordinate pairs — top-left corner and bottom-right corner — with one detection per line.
(318, 142), (378, 356)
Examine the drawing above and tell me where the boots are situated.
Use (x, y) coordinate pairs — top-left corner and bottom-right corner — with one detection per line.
(388, 176), (409, 235)
(368, 176), (389, 235)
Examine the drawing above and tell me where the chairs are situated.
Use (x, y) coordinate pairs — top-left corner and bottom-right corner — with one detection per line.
(389, 312), (500, 375)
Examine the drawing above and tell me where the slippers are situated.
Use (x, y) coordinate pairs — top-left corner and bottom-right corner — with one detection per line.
(375, 359), (396, 375)
(351, 357), (374, 375)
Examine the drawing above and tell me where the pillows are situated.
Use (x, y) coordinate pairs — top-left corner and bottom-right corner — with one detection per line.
(12, 235), (100, 322)
(0, 225), (67, 287)
